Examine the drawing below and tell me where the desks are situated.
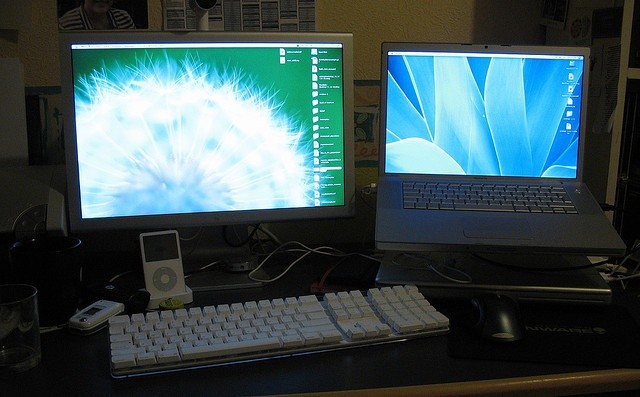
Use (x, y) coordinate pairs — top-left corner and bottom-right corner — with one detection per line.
(0, 217), (639, 397)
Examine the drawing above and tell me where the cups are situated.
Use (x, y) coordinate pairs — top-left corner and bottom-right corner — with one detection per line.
(10, 235), (83, 326)
(1, 284), (42, 372)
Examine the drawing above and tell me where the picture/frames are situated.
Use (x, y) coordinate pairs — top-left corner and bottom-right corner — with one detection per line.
(354, 80), (381, 167)
(26, 85), (64, 165)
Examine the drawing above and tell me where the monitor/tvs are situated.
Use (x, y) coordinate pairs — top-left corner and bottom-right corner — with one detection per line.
(58, 30), (356, 292)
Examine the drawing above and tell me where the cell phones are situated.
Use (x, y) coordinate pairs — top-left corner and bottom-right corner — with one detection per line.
(68, 299), (125, 332)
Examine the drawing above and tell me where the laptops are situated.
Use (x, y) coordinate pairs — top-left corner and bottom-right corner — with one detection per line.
(374, 41), (627, 256)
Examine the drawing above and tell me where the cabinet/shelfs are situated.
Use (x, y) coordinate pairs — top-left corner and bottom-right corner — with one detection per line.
(604, 0), (640, 246)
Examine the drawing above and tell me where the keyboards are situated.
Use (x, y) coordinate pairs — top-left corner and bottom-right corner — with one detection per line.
(107, 284), (450, 379)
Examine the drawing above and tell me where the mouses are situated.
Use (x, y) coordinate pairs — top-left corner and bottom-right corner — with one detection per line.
(471, 292), (524, 343)
